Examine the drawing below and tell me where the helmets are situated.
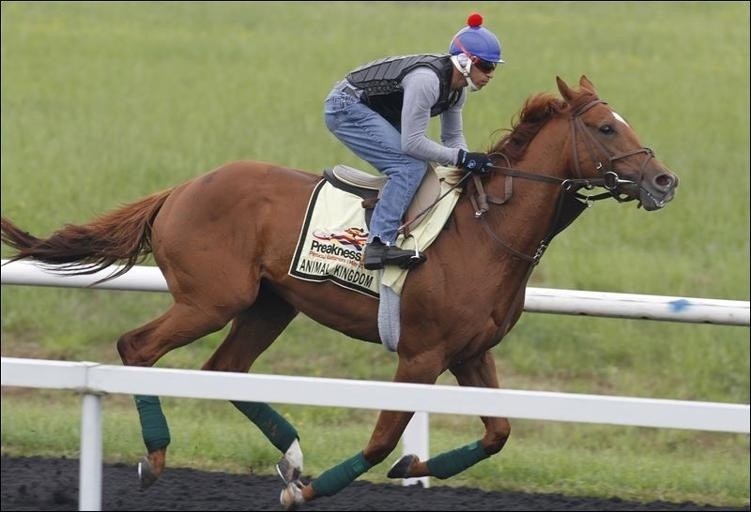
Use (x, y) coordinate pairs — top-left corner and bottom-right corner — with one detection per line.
(451, 25), (505, 67)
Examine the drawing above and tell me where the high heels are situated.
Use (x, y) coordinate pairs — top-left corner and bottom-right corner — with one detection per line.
(363, 239), (427, 270)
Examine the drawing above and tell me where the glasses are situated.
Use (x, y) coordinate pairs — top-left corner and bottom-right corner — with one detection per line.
(471, 56), (498, 74)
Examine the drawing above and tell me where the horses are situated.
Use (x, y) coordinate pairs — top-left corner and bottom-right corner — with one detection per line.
(1, 74), (679, 511)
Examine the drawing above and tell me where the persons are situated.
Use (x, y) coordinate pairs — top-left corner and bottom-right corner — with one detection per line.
(318, 10), (505, 272)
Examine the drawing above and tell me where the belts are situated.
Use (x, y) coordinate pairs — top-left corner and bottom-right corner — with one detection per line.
(333, 81), (361, 100)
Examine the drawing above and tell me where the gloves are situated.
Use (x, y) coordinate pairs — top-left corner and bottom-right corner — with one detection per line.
(456, 149), (490, 171)
